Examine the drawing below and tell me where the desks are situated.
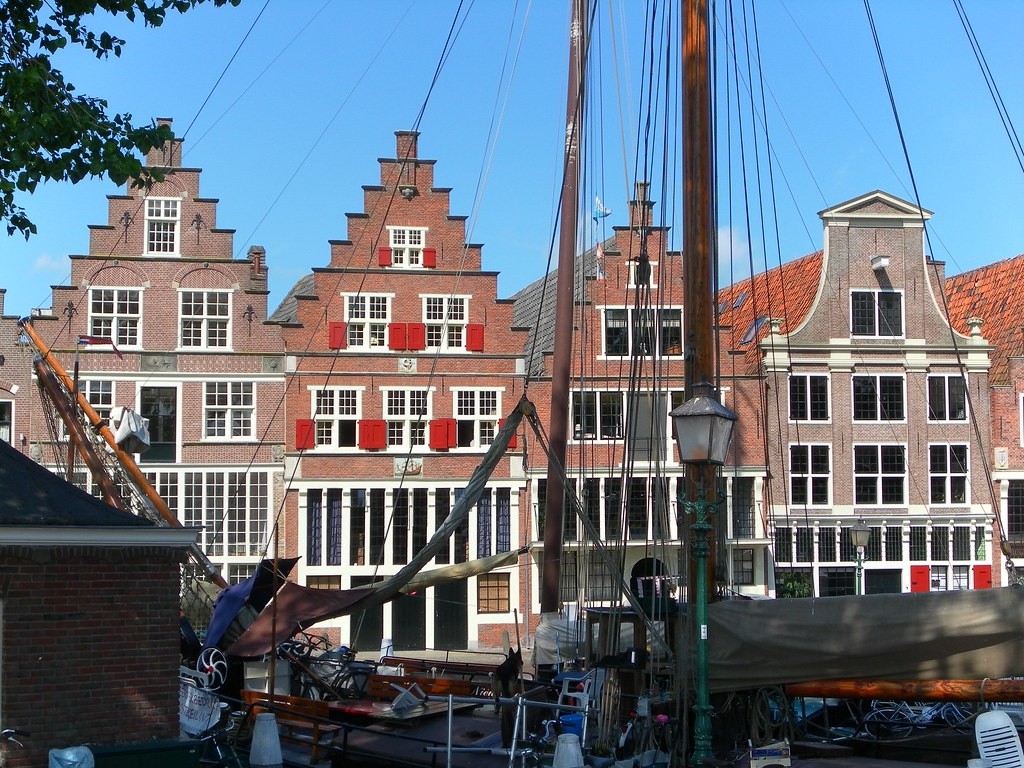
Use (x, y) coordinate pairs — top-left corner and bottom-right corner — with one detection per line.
(326, 698), (479, 721)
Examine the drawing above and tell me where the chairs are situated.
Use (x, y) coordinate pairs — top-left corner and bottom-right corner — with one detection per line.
(974, 711), (1024, 768)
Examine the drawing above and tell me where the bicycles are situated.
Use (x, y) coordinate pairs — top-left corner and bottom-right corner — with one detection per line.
(0, 728), (95, 768)
(863, 699), (975, 740)
(290, 629), (380, 701)
(616, 711), (647, 761)
(511, 719), (615, 768)
(201, 719), (243, 768)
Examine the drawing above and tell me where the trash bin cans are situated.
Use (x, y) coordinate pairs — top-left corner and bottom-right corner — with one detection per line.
(50, 738), (206, 768)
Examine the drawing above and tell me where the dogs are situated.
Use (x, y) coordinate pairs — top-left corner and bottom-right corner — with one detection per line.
(490, 646), (523, 715)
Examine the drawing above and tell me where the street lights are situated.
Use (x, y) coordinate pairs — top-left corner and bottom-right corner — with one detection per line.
(850, 515), (872, 595)
(668, 370), (738, 768)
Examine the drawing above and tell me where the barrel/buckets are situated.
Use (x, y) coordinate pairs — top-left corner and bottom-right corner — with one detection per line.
(560, 714), (583, 739)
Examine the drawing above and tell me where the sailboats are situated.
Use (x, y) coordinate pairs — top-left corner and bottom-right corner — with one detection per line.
(18, 1), (1023, 767)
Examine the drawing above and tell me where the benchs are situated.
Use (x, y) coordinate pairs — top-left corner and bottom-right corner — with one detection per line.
(239, 690), (349, 766)
(367, 673), (475, 716)
